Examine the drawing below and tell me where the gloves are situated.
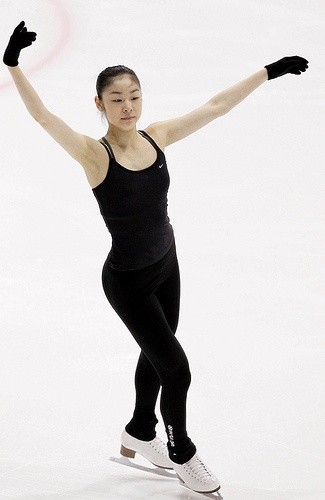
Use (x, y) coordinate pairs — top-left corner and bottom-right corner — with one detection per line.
(264, 56), (309, 80)
(3, 21), (37, 67)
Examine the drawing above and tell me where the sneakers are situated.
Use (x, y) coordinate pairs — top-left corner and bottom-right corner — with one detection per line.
(169, 450), (223, 500)
(109, 430), (177, 478)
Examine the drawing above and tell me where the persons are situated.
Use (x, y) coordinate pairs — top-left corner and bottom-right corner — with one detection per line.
(4, 20), (309, 494)
